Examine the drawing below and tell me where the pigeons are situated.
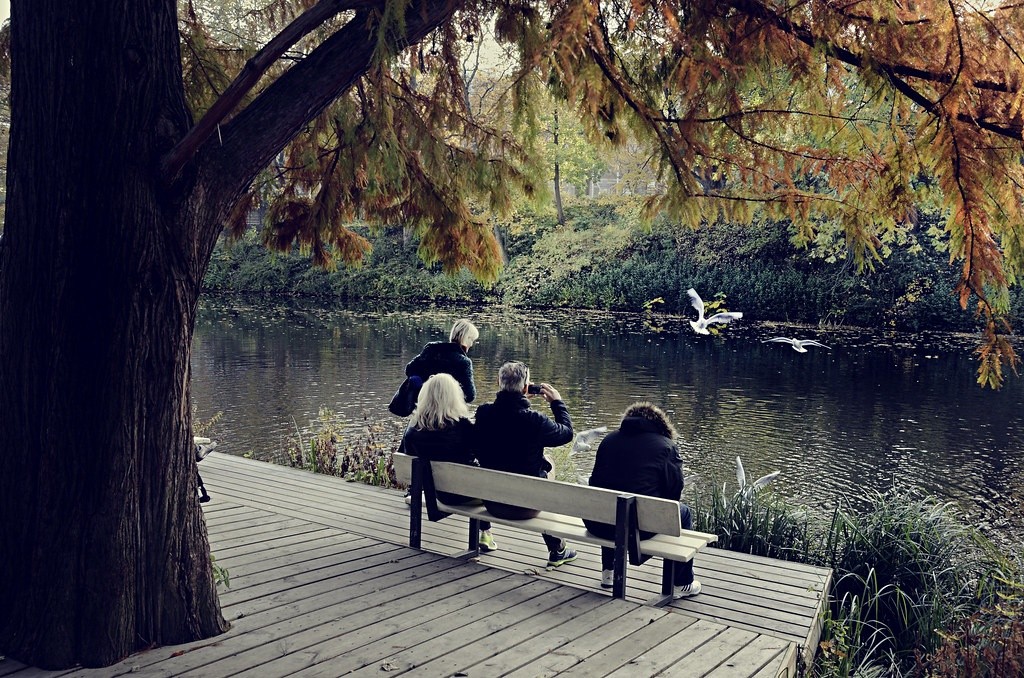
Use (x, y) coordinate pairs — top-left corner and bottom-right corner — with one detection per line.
(688, 288), (743, 335)
(569, 425), (607, 456)
(762, 337), (831, 353)
(735, 456), (780, 498)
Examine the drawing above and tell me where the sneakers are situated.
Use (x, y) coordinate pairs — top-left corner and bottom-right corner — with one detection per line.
(478, 532), (499, 552)
(673, 579), (702, 600)
(600, 569), (614, 588)
(546, 547), (578, 570)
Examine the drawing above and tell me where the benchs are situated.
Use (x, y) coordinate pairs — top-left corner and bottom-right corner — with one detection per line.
(393, 451), (719, 609)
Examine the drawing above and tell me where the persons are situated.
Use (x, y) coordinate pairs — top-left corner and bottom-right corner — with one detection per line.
(582, 401), (701, 599)
(405, 318), (480, 505)
(474, 360), (578, 567)
(396, 373), (498, 551)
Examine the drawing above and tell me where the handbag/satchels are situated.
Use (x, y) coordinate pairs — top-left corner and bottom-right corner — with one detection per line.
(388, 376), (425, 418)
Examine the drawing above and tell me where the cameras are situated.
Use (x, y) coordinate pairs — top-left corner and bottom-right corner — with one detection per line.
(528, 384), (544, 394)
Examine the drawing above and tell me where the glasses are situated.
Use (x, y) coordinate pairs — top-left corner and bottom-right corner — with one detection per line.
(504, 359), (529, 374)
